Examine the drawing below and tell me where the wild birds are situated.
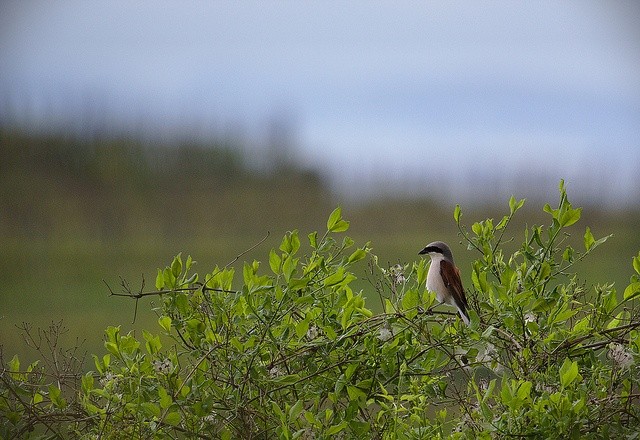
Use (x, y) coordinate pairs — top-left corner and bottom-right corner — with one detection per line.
(418, 241), (471, 326)
